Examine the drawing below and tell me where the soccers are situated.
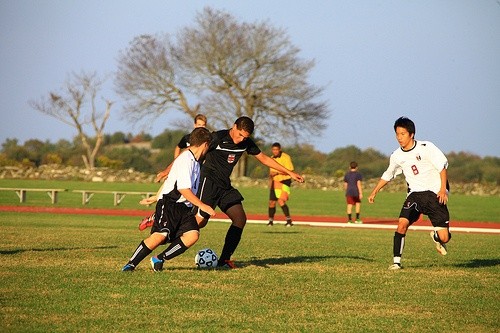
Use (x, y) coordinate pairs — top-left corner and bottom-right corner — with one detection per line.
(195, 248), (218, 270)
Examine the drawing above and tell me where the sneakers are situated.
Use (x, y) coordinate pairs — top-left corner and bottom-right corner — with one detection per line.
(387, 263), (402, 271)
(430, 230), (447, 256)
(138, 215), (153, 230)
(150, 255), (164, 271)
(218, 260), (236, 270)
(122, 262), (135, 272)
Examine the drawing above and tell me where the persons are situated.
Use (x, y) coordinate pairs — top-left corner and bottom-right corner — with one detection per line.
(343, 162), (364, 224)
(153, 116), (305, 268)
(264, 143), (294, 227)
(122, 127), (217, 271)
(368, 116), (452, 270)
(174, 114), (207, 158)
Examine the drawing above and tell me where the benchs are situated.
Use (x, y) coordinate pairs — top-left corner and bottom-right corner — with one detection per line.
(73, 188), (160, 208)
(0, 186), (68, 205)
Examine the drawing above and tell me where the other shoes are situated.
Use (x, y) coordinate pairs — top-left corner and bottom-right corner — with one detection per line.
(354, 219), (363, 224)
(346, 220), (353, 224)
(266, 221), (273, 226)
(285, 221), (292, 227)
